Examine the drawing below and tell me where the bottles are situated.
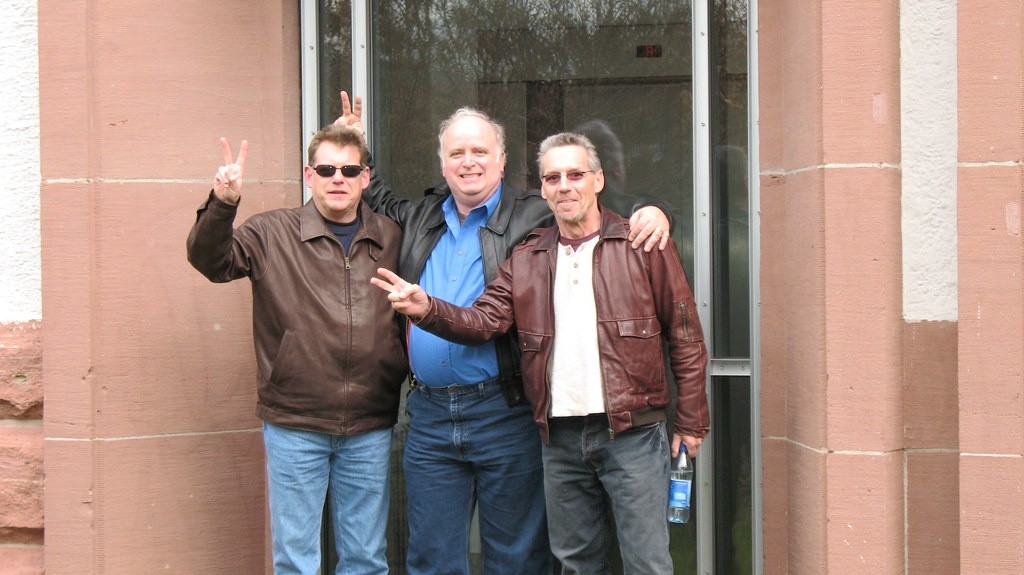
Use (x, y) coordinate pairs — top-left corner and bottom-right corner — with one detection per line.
(665, 443), (693, 523)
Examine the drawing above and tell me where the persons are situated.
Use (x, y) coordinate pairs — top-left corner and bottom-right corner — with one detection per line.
(186, 123), (405, 575)
(569, 122), (750, 575)
(333, 91), (677, 575)
(369, 132), (711, 575)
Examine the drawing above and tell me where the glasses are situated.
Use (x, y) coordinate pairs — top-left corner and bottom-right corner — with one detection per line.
(542, 170), (594, 184)
(314, 165), (364, 178)
